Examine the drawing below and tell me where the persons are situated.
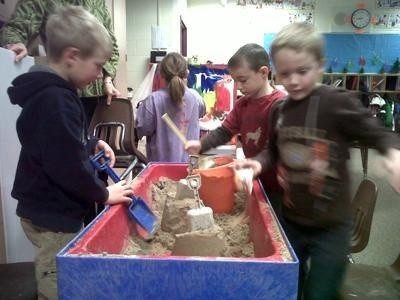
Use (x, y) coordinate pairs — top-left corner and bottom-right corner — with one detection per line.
(184, 43), (288, 215)
(133, 52), (206, 164)
(7, 5), (134, 300)
(232, 22), (400, 300)
(1, 0), (120, 227)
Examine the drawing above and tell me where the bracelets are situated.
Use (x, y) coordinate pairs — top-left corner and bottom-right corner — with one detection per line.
(103, 80), (113, 85)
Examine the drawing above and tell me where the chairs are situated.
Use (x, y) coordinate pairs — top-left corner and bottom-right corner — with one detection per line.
(78, 94), (149, 220)
(322, 177), (400, 300)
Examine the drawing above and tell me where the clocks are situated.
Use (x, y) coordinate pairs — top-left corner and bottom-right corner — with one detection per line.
(351, 8), (370, 28)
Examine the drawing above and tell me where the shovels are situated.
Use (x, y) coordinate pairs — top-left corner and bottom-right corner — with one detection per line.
(89, 150), (161, 235)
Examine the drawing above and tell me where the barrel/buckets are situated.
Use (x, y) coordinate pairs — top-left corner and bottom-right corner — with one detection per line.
(186, 157), (238, 214)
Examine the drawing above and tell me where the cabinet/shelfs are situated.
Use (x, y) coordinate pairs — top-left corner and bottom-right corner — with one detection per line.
(271, 73), (400, 101)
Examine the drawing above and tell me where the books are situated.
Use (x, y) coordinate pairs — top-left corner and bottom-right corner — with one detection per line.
(333, 76), (385, 91)
